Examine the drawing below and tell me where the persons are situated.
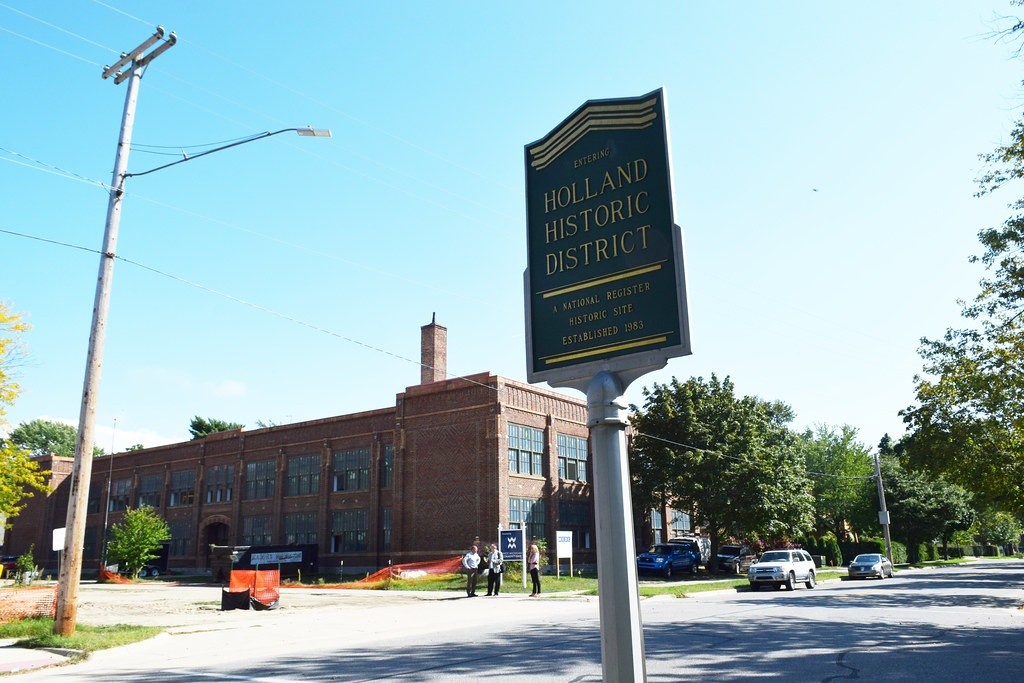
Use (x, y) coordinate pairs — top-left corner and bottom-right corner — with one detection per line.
(462, 545), (480, 597)
(483, 544), (506, 597)
(528, 545), (541, 598)
(472, 536), (482, 552)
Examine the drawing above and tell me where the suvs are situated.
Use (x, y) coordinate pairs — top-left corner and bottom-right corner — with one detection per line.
(706, 545), (758, 574)
(747, 549), (816, 590)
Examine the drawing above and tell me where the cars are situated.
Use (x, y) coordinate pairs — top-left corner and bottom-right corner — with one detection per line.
(848, 554), (893, 579)
(636, 533), (711, 578)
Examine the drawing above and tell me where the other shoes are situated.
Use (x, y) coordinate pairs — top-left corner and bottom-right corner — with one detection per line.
(484, 593), (492, 596)
(529, 593), (536, 597)
(468, 594), (478, 597)
(494, 593), (498, 596)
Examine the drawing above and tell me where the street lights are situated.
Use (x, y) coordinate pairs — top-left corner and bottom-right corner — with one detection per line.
(53, 124), (331, 638)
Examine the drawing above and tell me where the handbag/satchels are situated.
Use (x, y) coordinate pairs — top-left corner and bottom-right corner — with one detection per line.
(498, 552), (506, 573)
